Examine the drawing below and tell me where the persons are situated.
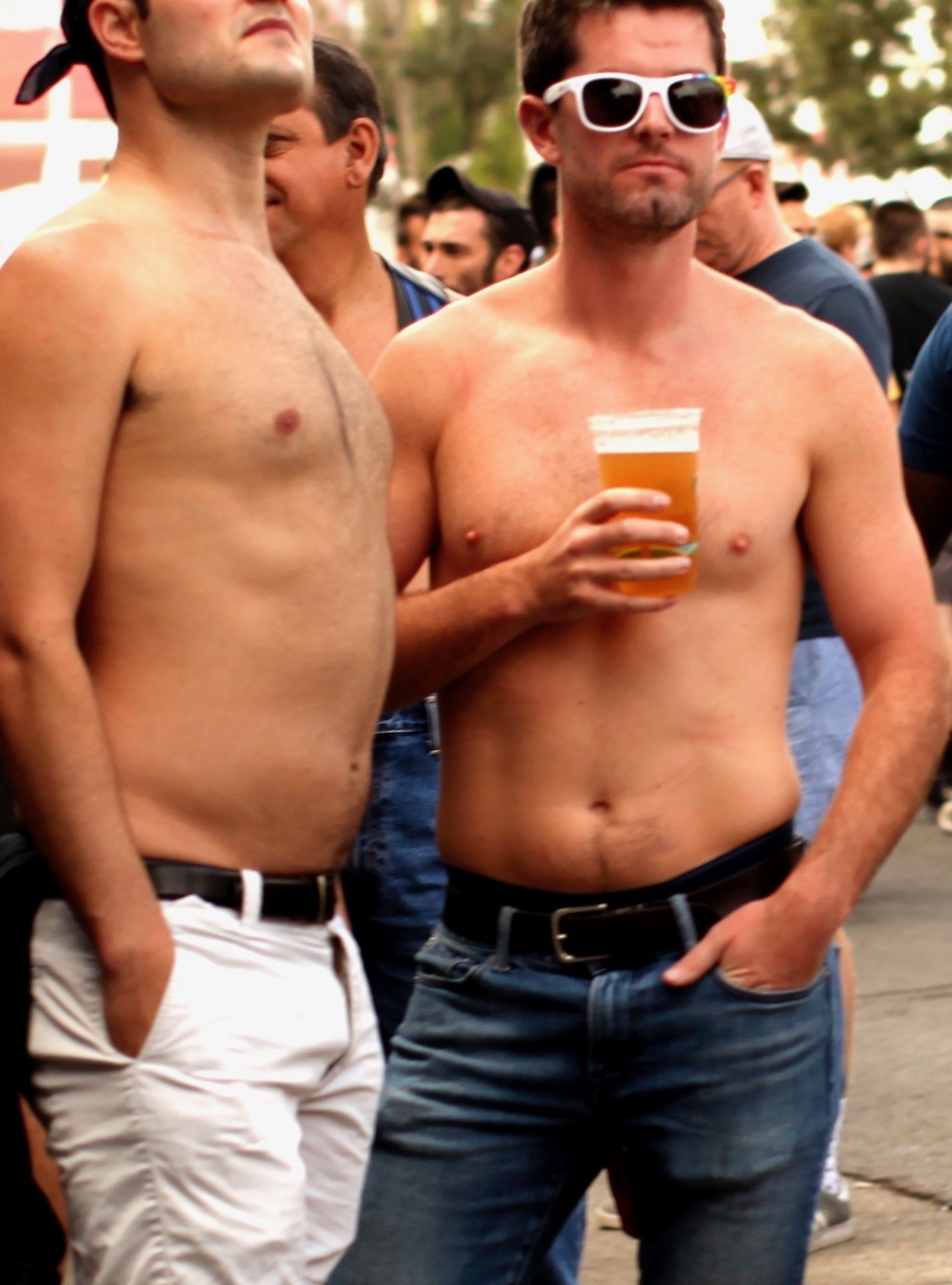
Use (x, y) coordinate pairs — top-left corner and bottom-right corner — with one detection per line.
(326, 0), (950, 1285)
(0, 0), (952, 1285)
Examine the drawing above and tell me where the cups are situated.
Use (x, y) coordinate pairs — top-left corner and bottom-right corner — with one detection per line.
(585, 408), (703, 599)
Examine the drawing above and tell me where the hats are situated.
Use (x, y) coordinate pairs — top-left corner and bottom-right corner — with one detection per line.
(426, 164), (541, 243)
(722, 92), (775, 163)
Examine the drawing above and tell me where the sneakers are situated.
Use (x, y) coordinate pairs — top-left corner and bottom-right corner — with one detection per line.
(806, 1175), (857, 1255)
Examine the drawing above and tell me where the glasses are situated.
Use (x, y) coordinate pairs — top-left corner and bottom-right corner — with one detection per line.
(541, 71), (738, 134)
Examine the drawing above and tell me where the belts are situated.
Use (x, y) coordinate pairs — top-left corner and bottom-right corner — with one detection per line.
(39, 857), (339, 926)
(442, 834), (809, 975)
